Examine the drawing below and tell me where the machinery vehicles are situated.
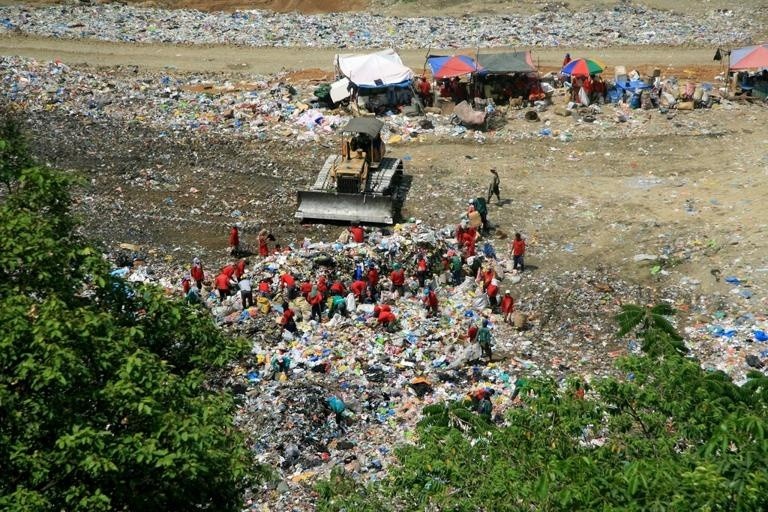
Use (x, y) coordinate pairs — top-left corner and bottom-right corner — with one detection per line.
(289, 115), (413, 226)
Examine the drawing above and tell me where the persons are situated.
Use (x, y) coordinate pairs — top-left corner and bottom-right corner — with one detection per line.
(575, 383), (584, 399)
(326, 394), (344, 424)
(472, 320), (493, 362)
(181, 196), (527, 331)
(485, 168), (501, 204)
(272, 355), (290, 373)
(467, 389), (495, 416)
(419, 53), (606, 107)
